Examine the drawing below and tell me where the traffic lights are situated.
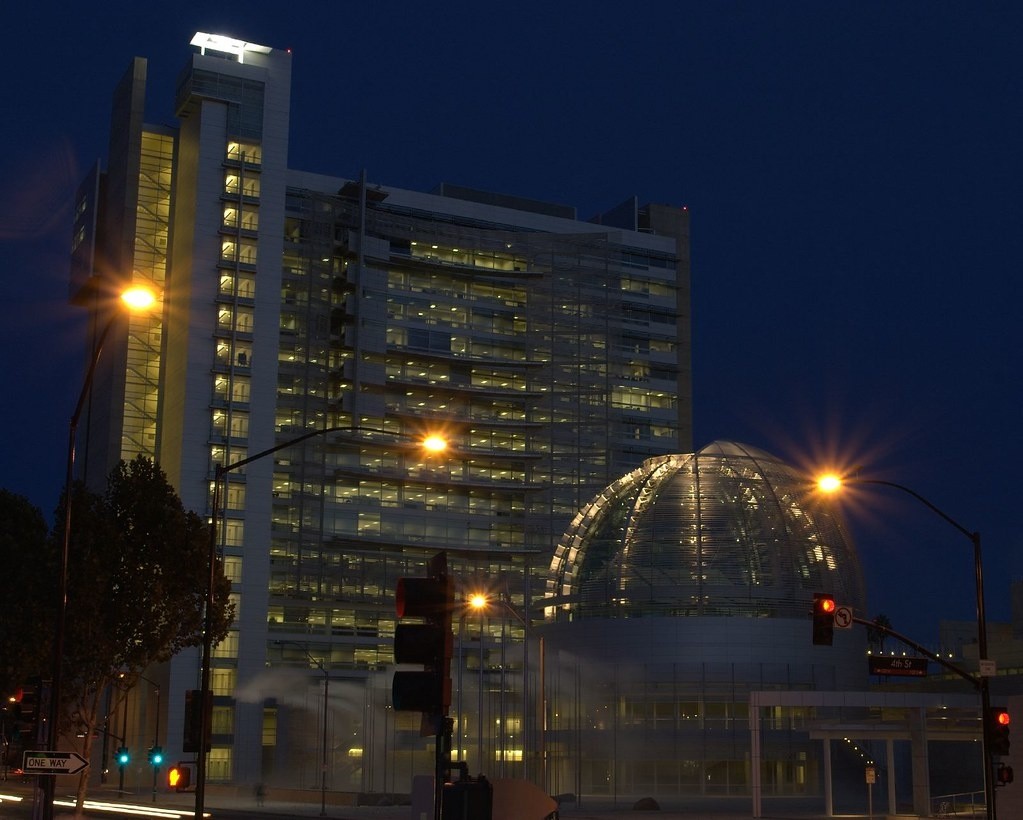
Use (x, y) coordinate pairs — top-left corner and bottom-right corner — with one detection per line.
(114, 747), (129, 766)
(997, 766), (1014, 784)
(812, 591), (836, 647)
(391, 549), (455, 720)
(989, 705), (1011, 757)
(147, 745), (162, 766)
(165, 766), (191, 789)
(441, 775), (494, 820)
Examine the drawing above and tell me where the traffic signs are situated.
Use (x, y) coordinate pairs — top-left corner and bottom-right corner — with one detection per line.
(21, 751), (92, 776)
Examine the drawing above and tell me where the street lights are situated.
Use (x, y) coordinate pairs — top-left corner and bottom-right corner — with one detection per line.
(92, 678), (130, 796)
(117, 671), (161, 802)
(815, 471), (998, 820)
(273, 639), (329, 817)
(191, 426), (453, 820)
(468, 593), (548, 793)
(40, 286), (160, 820)
